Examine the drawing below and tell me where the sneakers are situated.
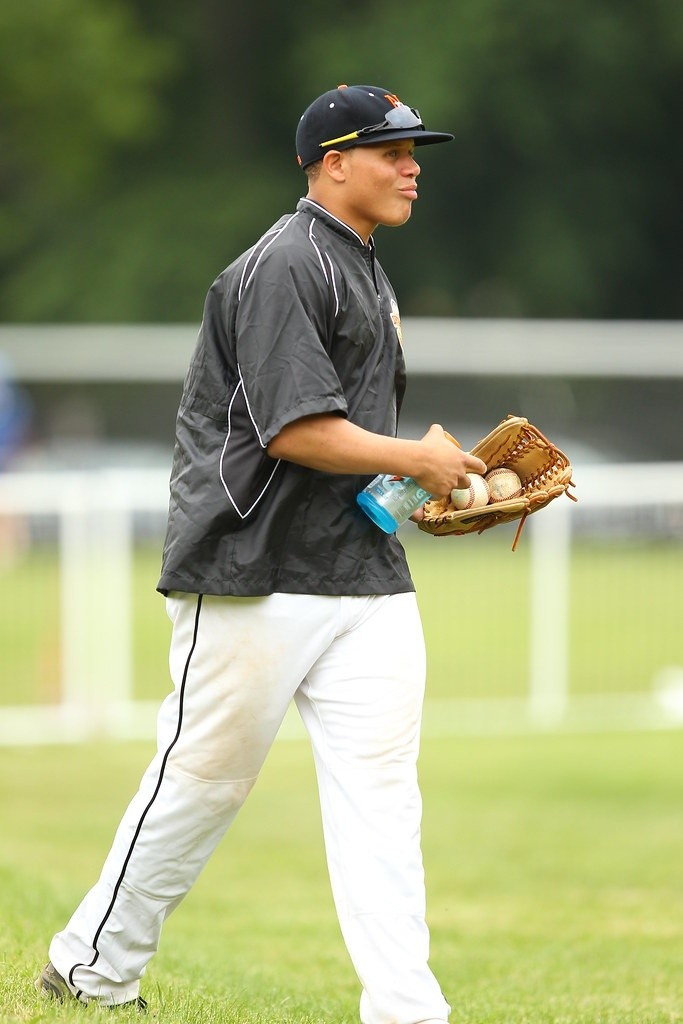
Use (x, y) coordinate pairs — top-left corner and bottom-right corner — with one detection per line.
(35, 960), (147, 1014)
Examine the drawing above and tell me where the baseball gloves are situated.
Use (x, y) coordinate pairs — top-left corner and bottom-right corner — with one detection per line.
(415, 411), (579, 539)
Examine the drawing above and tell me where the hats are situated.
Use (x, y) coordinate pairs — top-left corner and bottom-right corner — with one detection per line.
(295, 85), (455, 169)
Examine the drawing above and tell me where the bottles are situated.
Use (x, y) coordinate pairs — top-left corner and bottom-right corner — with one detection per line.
(356, 429), (461, 536)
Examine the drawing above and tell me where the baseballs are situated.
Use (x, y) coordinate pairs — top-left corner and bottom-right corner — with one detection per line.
(483, 466), (523, 505)
(449, 471), (492, 511)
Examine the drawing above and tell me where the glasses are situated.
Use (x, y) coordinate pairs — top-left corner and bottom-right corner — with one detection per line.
(317, 106), (426, 148)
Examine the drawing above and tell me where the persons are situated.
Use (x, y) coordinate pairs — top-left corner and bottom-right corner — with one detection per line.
(33, 85), (488, 1024)
